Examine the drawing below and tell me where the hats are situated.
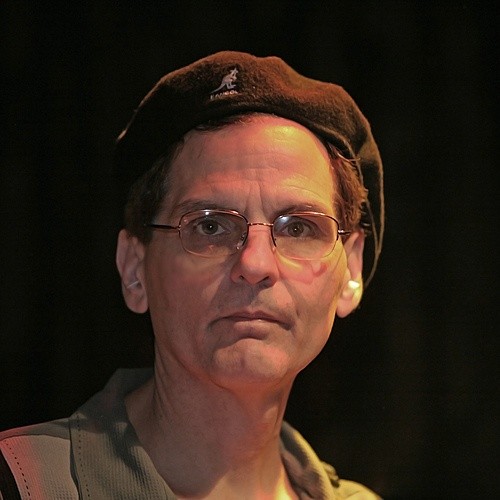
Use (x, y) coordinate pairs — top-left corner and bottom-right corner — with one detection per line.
(112, 48), (386, 291)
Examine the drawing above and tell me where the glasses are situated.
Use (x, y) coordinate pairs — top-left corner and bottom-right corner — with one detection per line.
(142, 207), (352, 262)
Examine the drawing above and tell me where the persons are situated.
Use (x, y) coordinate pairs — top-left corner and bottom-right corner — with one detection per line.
(2, 51), (387, 499)
